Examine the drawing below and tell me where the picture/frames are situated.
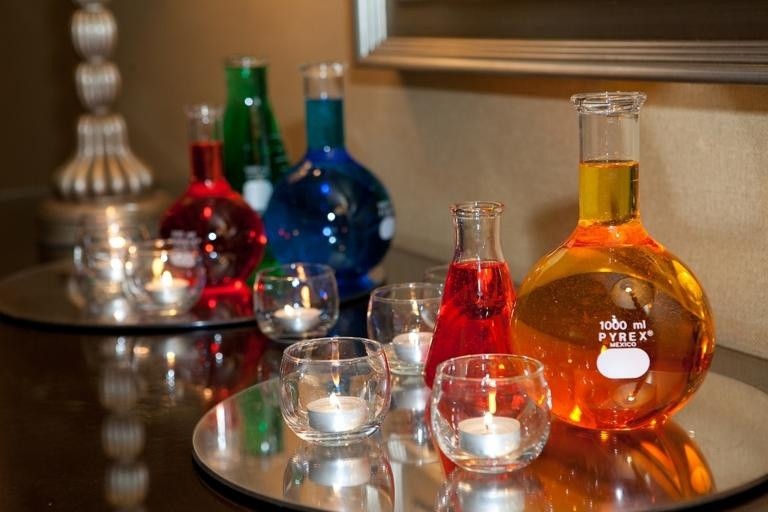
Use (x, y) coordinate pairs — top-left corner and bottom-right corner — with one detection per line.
(347, 1), (766, 86)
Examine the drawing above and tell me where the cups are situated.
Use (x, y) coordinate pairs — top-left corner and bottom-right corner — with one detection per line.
(382, 373), (441, 465)
(252, 264), (339, 344)
(281, 443), (394, 512)
(366, 265), (451, 375)
(72, 210), (205, 321)
(277, 337), (391, 446)
(428, 353), (551, 474)
(82, 329), (203, 412)
(432, 468), (554, 512)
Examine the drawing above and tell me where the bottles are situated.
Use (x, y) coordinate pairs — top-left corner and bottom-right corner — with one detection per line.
(159, 105), (265, 304)
(262, 60), (396, 303)
(211, 54), (289, 214)
(422, 201), (516, 392)
(519, 410), (714, 510)
(201, 325), (279, 457)
(509, 92), (714, 432)
